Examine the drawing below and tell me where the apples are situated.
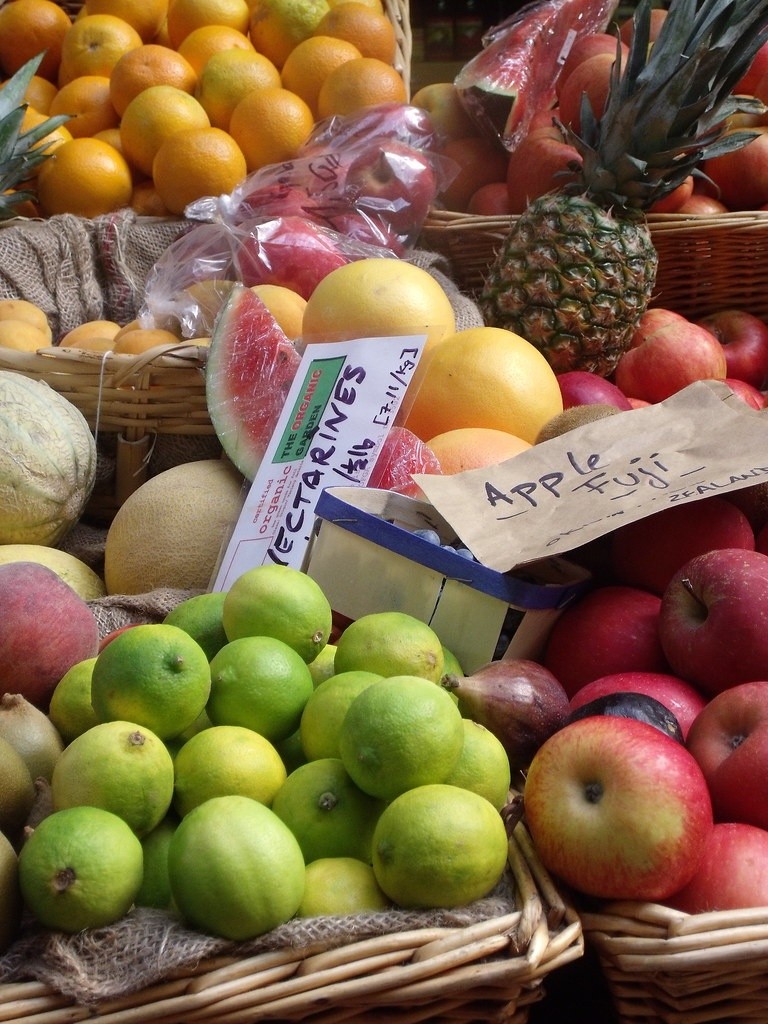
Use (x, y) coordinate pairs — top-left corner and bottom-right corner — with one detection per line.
(174, 104), (440, 296)
(523, 306), (768, 915)
(409, 9), (768, 220)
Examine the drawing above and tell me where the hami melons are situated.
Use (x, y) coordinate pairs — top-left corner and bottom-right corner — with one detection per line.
(0, 372), (246, 598)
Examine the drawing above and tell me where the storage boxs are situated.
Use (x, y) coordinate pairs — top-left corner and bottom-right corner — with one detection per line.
(301, 487), (589, 676)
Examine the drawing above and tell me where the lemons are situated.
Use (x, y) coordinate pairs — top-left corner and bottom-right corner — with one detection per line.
(19, 562), (512, 945)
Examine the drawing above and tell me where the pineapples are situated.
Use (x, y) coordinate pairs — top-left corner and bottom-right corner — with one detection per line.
(484, 0), (768, 379)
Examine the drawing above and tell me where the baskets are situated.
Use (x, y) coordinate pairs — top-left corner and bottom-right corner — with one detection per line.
(0, 0), (413, 231)
(418, 210), (768, 331)
(0, 788), (586, 1024)
(0, 221), (485, 436)
(567, 901), (768, 1024)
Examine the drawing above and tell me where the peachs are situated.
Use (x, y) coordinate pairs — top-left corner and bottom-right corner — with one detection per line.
(0, 562), (100, 711)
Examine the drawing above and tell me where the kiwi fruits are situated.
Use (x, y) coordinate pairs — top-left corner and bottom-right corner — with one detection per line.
(533, 404), (620, 443)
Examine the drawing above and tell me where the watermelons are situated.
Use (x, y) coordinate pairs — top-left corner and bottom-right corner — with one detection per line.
(206, 281), (446, 503)
(454, 0), (622, 152)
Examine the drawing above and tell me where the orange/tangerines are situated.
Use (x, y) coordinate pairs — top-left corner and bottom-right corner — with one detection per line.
(0, 0), (407, 208)
(0, 258), (562, 485)
